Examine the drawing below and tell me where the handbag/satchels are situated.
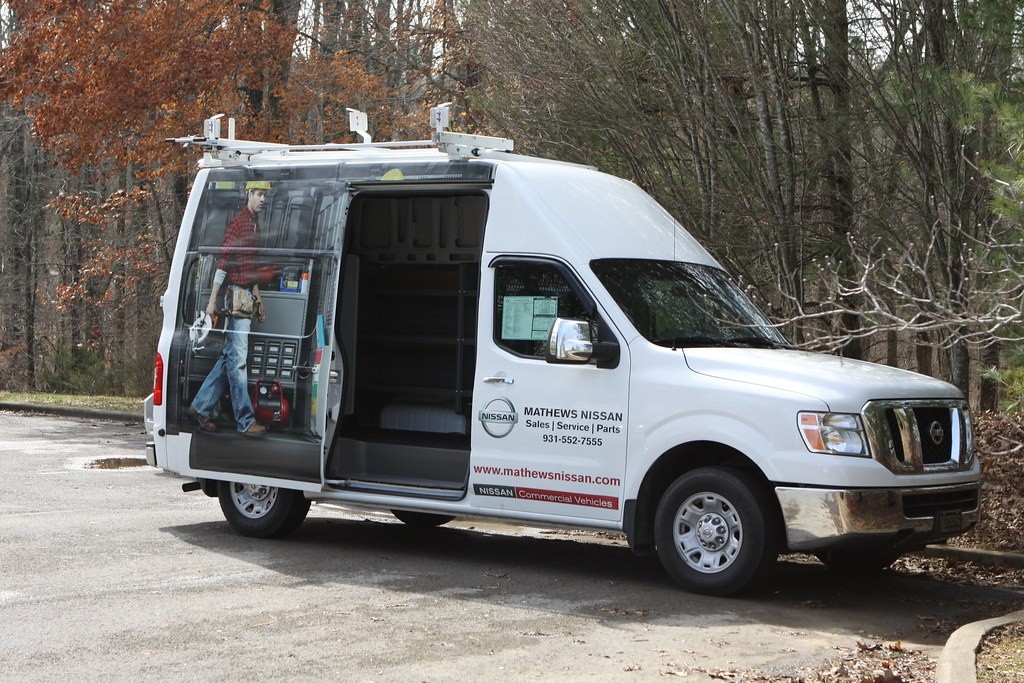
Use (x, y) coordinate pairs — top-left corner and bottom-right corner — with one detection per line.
(225, 286), (259, 319)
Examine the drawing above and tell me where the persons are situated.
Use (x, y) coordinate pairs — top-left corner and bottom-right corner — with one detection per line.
(187, 180), (274, 440)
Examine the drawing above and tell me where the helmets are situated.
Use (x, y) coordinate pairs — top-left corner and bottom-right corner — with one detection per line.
(246, 180), (272, 192)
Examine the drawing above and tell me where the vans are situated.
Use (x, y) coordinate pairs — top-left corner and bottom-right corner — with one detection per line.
(145, 105), (983, 596)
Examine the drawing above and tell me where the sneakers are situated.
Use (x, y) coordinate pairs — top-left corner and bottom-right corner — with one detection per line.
(238, 423), (268, 439)
(188, 406), (217, 431)
